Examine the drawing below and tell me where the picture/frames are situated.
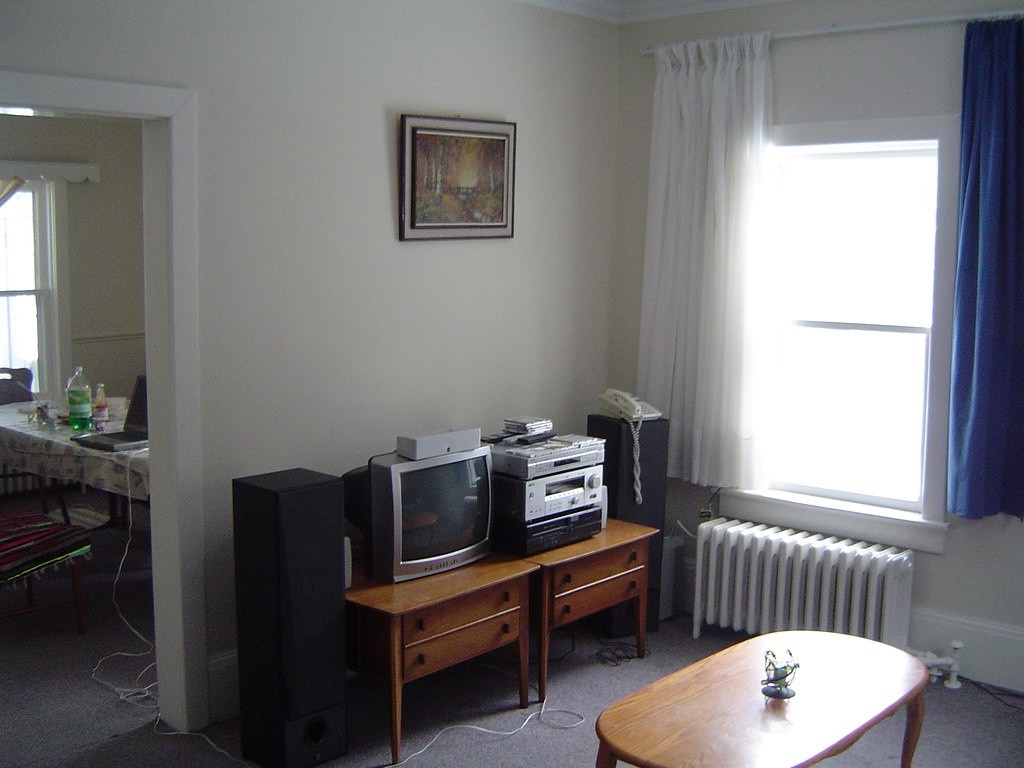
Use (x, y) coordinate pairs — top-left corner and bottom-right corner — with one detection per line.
(398, 113), (517, 242)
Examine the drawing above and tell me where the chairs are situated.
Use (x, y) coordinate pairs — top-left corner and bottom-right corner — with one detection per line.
(0, 368), (72, 527)
(0, 473), (94, 635)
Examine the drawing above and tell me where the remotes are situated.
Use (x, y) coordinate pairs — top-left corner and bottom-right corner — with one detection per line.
(70, 432), (91, 441)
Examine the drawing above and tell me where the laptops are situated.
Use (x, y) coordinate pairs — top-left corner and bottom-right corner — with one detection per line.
(77, 374), (148, 452)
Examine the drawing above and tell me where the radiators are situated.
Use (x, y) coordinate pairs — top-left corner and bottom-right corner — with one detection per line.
(692, 517), (964, 691)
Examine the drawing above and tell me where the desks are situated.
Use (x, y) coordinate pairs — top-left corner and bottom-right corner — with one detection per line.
(523, 517), (660, 704)
(347, 552), (542, 764)
(596, 630), (930, 768)
(0, 399), (150, 505)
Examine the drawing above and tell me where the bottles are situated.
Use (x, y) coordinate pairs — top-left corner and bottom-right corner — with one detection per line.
(67, 367), (93, 431)
(94, 384), (108, 431)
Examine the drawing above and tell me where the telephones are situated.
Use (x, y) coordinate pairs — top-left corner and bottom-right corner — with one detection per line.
(597, 388), (663, 423)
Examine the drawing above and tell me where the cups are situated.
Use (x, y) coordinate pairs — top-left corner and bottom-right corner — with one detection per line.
(36, 400), (49, 423)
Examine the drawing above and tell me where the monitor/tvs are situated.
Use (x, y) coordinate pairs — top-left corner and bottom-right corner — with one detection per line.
(340, 445), (494, 583)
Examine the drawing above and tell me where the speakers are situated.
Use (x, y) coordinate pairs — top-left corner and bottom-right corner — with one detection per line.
(586, 414), (670, 640)
(231, 468), (350, 768)
